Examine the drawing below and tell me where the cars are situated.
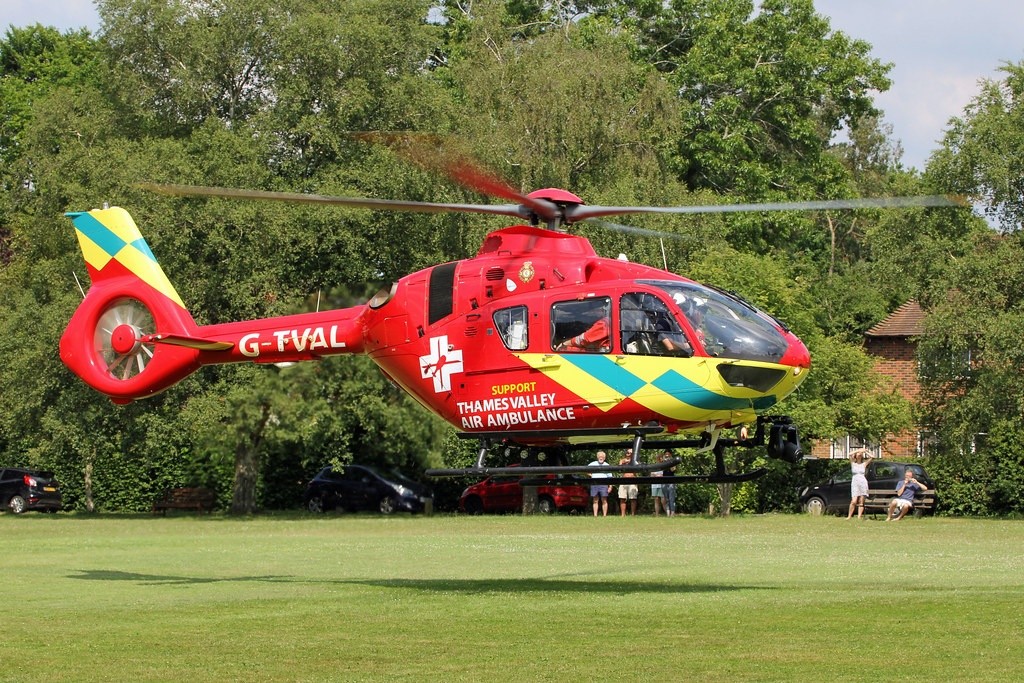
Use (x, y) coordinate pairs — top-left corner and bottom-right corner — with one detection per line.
(302, 458), (436, 514)
(458, 463), (590, 514)
(802, 459), (937, 518)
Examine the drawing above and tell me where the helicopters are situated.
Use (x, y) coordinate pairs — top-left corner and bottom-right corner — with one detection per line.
(58, 128), (984, 498)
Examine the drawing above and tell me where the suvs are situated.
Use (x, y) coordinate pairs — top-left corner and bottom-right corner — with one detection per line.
(0, 467), (63, 515)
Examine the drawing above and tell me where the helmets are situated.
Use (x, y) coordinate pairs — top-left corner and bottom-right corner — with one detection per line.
(685, 295), (709, 330)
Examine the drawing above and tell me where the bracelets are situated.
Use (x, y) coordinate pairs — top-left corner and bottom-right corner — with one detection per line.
(562, 342), (564, 346)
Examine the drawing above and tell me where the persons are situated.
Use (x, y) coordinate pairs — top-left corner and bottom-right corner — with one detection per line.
(846, 449), (873, 520)
(587, 451), (614, 516)
(885, 470), (928, 522)
(651, 448), (676, 518)
(619, 449), (638, 517)
(552, 292), (712, 354)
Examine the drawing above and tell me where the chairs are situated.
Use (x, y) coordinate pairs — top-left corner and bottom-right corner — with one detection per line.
(882, 470), (893, 479)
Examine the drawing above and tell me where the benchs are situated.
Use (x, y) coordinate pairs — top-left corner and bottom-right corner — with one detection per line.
(865, 489), (936, 520)
